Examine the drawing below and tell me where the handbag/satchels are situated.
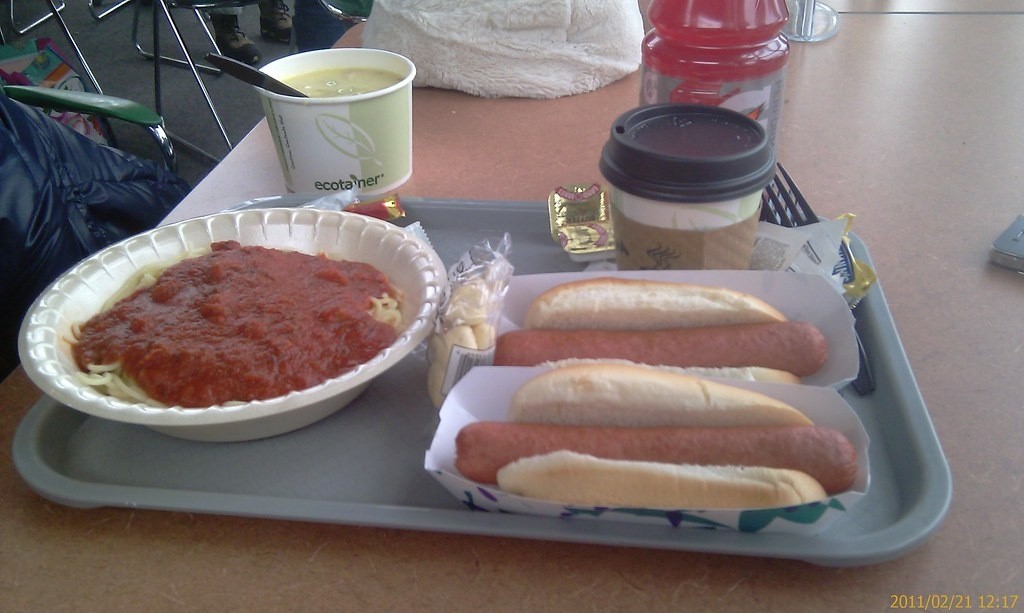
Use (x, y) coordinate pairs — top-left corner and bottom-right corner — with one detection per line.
(0, 37), (114, 146)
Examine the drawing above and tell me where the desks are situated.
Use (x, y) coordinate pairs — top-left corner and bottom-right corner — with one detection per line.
(0, 0), (1024, 613)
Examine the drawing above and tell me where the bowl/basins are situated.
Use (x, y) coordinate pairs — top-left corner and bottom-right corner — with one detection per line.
(18, 206), (446, 446)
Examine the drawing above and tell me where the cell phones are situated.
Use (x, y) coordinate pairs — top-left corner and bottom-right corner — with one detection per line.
(990, 215), (1024, 271)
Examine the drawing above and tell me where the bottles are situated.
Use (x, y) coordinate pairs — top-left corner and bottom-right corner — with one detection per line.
(638, 0), (791, 160)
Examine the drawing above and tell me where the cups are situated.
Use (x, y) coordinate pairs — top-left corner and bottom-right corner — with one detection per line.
(598, 102), (777, 272)
(253, 46), (417, 195)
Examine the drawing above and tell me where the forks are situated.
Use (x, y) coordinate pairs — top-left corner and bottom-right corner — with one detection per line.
(762, 162), (876, 397)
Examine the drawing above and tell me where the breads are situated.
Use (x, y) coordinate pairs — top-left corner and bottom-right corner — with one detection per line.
(426, 280), (495, 407)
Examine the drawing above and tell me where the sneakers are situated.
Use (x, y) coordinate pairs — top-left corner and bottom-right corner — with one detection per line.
(258, 0), (293, 39)
(209, 14), (262, 63)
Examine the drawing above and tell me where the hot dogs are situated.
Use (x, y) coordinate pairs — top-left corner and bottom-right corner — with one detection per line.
(493, 278), (825, 381)
(457, 363), (856, 510)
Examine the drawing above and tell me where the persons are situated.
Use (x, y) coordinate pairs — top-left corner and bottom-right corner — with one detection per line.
(206, 0), (293, 65)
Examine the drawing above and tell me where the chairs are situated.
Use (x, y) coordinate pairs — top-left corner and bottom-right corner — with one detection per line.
(0, 0), (269, 174)
